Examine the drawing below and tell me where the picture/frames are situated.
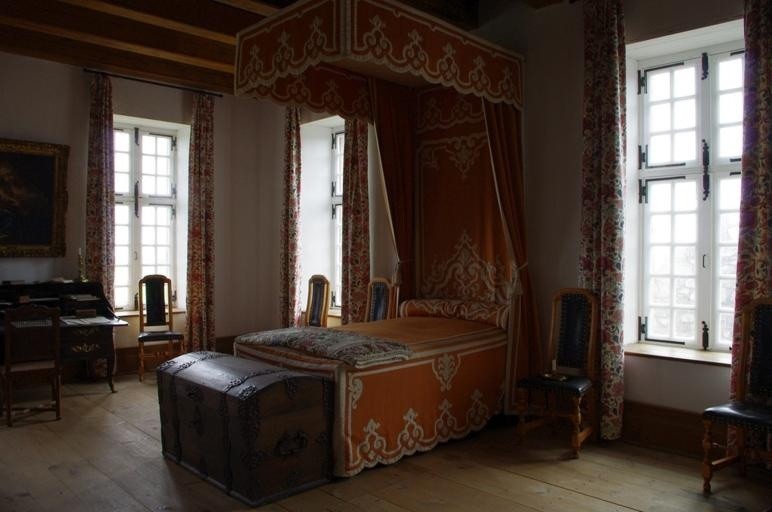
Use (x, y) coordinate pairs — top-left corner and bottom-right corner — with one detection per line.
(0, 137), (70, 256)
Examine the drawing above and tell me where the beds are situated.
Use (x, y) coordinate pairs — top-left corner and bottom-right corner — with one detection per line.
(232, 0), (543, 477)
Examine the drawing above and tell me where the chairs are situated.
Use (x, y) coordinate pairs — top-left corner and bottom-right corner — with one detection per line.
(701, 298), (772, 496)
(305, 275), (330, 328)
(137, 274), (184, 382)
(364, 277), (391, 322)
(0, 305), (61, 428)
(514, 289), (598, 459)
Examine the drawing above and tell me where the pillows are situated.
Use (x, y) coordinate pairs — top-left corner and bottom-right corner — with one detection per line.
(400, 299), (509, 329)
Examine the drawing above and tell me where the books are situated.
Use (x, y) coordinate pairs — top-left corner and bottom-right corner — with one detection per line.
(48, 277), (74, 283)
(66, 294), (102, 301)
(2, 279), (25, 285)
(81, 316), (111, 324)
